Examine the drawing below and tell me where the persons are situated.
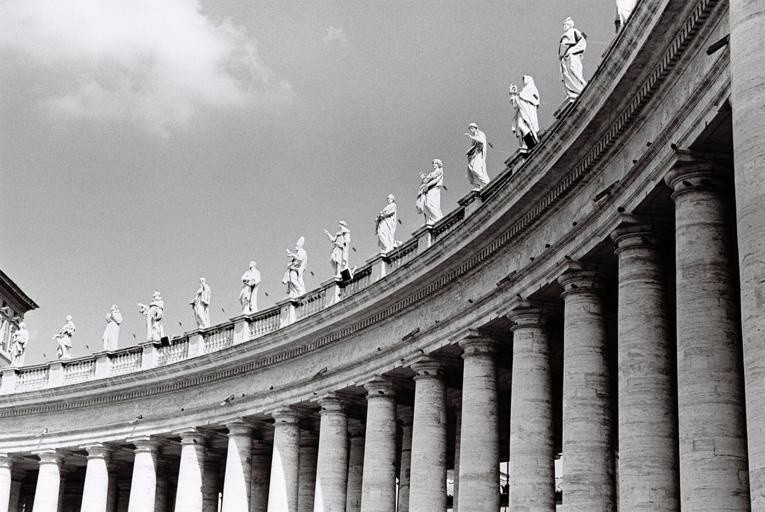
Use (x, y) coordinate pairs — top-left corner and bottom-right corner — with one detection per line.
(374, 193), (397, 254)
(415, 158), (448, 225)
(239, 260), (261, 315)
(53, 314), (75, 360)
(281, 237), (307, 296)
(558, 18), (587, 98)
(188, 277), (211, 328)
(102, 304), (122, 351)
(324, 220), (351, 278)
(138, 291), (164, 342)
(510, 75), (540, 148)
(464, 123), (492, 190)
(8, 321), (28, 367)
